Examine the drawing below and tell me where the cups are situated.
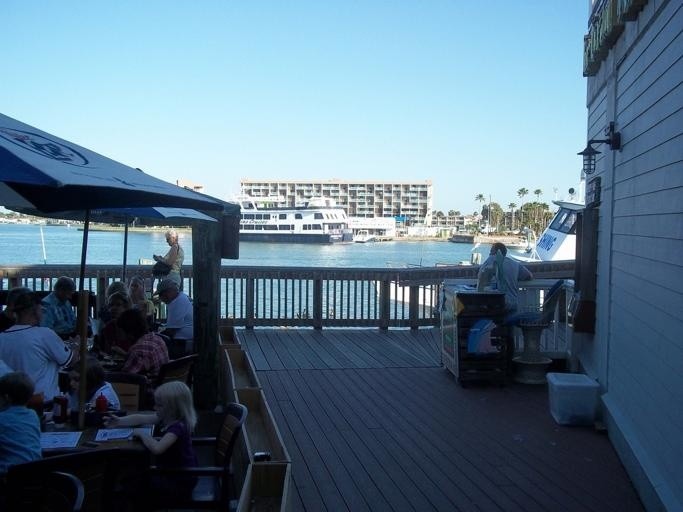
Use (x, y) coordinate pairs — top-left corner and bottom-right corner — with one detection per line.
(52, 395), (68, 425)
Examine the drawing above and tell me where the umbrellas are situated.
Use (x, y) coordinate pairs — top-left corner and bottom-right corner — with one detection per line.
(0, 108), (223, 292)
(4, 205), (219, 282)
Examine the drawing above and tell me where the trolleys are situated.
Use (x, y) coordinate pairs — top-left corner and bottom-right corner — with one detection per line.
(435, 278), (505, 388)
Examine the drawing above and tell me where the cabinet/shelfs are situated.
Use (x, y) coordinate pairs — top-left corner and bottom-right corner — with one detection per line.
(441, 286), (511, 383)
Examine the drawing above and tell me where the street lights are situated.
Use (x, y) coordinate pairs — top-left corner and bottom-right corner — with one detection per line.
(488, 194), (491, 236)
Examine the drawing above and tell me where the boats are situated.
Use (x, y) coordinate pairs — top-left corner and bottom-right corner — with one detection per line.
(501, 235), (531, 250)
(373, 235), (391, 241)
(370, 167), (584, 311)
(447, 235), (476, 243)
(352, 230), (372, 243)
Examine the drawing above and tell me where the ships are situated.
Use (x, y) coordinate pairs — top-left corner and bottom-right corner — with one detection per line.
(224, 194), (353, 244)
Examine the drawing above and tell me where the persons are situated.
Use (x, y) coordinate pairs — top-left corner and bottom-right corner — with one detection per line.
(480, 243), (533, 357)
(0, 277), (199, 499)
(153, 230), (184, 292)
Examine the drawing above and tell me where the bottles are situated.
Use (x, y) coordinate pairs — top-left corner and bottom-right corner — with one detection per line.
(95, 392), (107, 413)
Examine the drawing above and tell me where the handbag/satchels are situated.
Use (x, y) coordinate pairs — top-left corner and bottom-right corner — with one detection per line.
(152, 261), (171, 277)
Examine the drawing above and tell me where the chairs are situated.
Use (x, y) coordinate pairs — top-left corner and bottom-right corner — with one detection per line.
(5, 328), (248, 511)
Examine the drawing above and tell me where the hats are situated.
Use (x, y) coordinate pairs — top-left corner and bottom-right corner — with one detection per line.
(152, 279), (179, 296)
(13, 292), (51, 311)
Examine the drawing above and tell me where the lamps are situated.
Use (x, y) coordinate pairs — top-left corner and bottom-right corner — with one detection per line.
(578, 133), (620, 174)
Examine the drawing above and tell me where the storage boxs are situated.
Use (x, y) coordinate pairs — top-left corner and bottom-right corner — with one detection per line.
(546, 373), (601, 427)
